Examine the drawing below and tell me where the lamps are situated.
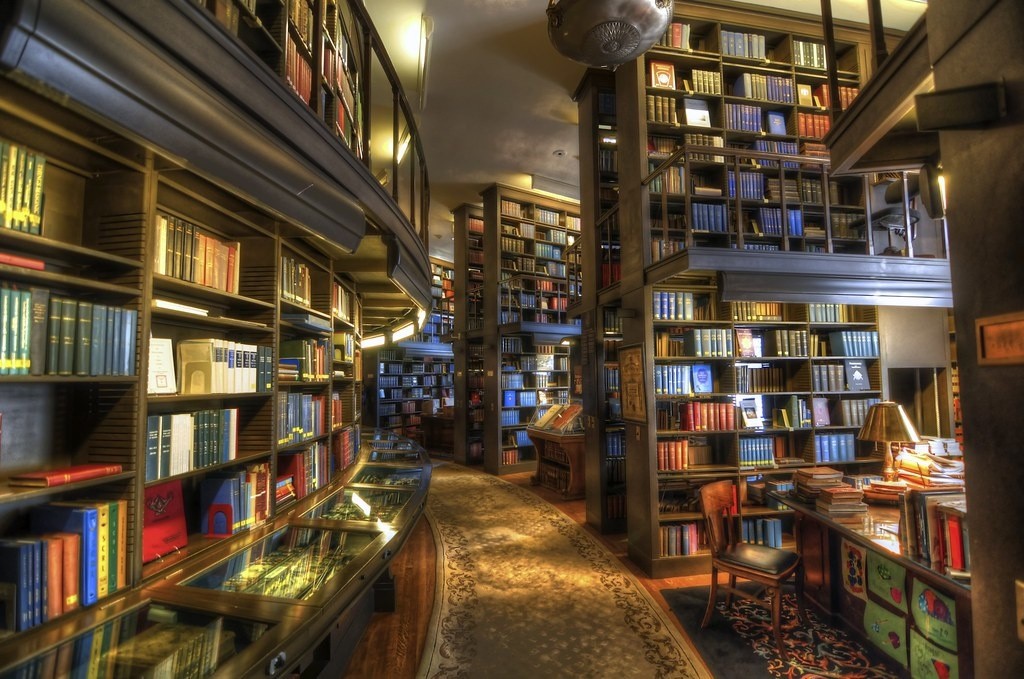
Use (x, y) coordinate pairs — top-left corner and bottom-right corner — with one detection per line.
(856, 401), (923, 482)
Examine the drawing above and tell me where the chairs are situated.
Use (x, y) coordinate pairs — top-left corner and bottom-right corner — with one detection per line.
(699, 480), (814, 662)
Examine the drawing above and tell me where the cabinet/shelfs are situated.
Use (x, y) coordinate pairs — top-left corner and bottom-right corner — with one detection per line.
(362, 254), (455, 448)
(449, 182), (581, 476)
(0, 0), (434, 679)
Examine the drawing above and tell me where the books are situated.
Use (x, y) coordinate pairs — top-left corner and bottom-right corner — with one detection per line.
(593, 19), (973, 576)
(15, 611), (139, 678)
(0, 0), (365, 631)
(469, 199), (582, 464)
(377, 263), (456, 433)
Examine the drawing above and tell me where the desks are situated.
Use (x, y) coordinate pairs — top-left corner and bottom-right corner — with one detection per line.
(768, 488), (971, 679)
(572, 0), (955, 578)
(420, 412), (455, 456)
